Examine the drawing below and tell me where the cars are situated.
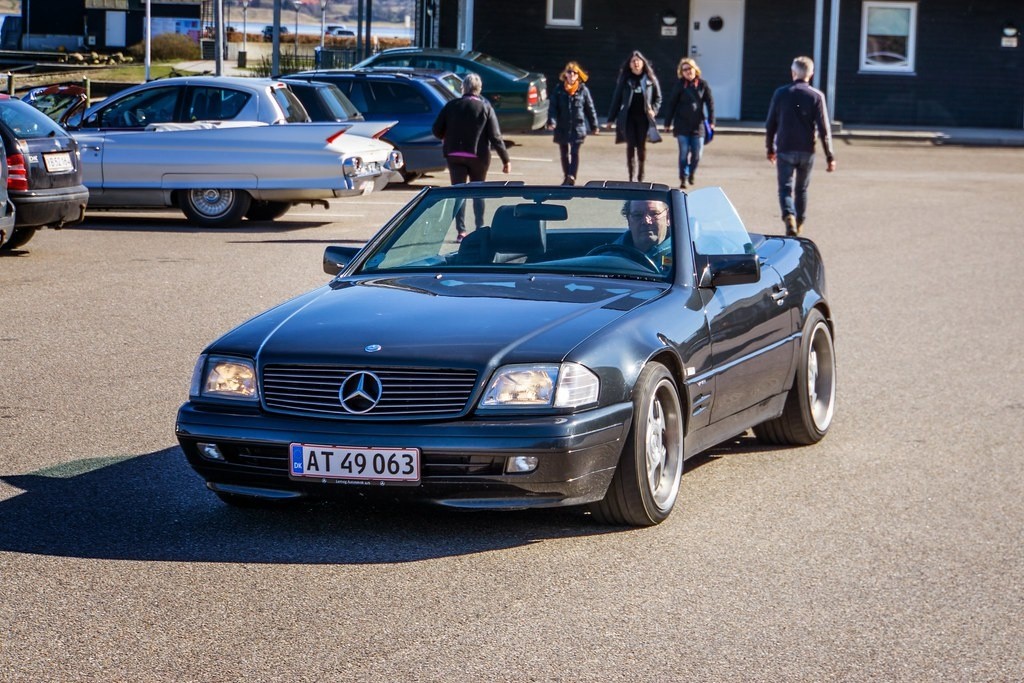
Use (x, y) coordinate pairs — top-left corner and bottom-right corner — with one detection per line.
(333, 28), (354, 36)
(53, 72), (310, 125)
(261, 25), (288, 41)
(0, 93), (88, 249)
(348, 46), (552, 133)
(324, 24), (344, 34)
(0, 134), (16, 248)
(175, 178), (838, 529)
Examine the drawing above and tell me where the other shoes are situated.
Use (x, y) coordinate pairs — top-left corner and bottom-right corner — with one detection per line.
(457, 232), (467, 243)
(688, 180), (693, 184)
(681, 184), (685, 188)
(564, 175), (575, 186)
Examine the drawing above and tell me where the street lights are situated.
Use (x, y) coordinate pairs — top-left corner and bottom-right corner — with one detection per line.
(318, 0), (328, 49)
(290, 0), (306, 55)
(238, 0), (252, 51)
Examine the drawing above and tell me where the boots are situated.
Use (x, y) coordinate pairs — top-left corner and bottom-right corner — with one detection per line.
(784, 214), (797, 236)
(796, 218), (802, 233)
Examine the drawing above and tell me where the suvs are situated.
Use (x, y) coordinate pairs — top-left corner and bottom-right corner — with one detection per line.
(275, 65), (461, 101)
(275, 68), (457, 175)
(282, 73), (365, 122)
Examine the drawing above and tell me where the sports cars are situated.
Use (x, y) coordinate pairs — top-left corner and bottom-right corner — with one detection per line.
(17, 85), (403, 229)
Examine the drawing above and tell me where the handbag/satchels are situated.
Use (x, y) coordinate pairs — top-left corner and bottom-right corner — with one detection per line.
(704, 119), (714, 145)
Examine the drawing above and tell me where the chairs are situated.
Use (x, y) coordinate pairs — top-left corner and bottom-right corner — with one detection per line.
(192, 92), (206, 119)
(457, 205), (546, 265)
(208, 92), (222, 117)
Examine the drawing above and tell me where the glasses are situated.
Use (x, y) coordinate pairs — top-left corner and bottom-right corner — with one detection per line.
(682, 67), (692, 71)
(630, 208), (667, 220)
(567, 70), (578, 74)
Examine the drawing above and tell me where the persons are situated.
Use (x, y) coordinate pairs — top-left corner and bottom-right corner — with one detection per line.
(598, 199), (672, 274)
(664, 58), (716, 189)
(764, 56), (836, 236)
(546, 62), (599, 186)
(432, 73), (510, 244)
(606, 50), (662, 183)
